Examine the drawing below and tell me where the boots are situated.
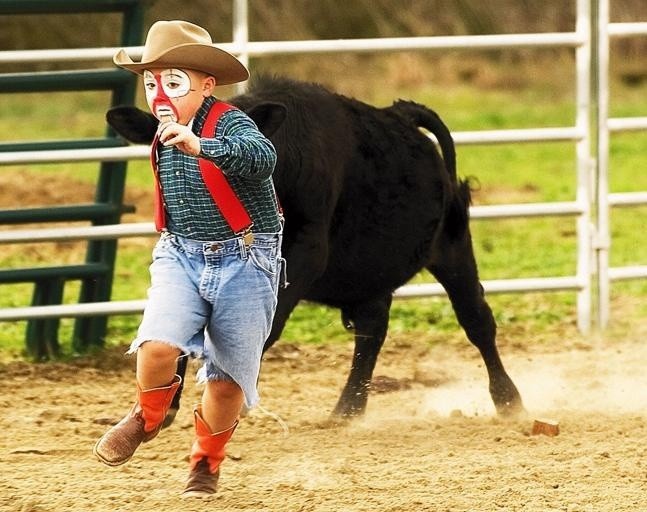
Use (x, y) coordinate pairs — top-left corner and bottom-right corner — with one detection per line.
(92, 374), (182, 467)
(182, 401), (239, 500)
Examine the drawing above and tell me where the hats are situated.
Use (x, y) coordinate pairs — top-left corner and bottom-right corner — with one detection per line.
(113, 20), (250, 86)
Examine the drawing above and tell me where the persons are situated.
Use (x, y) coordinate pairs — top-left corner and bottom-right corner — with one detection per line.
(93, 20), (288, 503)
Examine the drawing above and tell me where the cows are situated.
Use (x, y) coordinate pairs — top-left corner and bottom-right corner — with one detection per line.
(106, 65), (528, 418)
